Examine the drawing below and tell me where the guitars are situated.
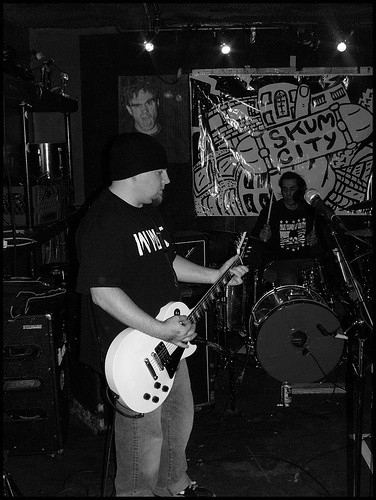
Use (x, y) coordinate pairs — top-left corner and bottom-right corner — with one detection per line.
(103, 231), (254, 415)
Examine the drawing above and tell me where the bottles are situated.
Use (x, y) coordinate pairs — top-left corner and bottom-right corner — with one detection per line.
(96, 402), (106, 435)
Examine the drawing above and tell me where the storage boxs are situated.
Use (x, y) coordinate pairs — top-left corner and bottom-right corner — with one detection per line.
(2, 181), (65, 227)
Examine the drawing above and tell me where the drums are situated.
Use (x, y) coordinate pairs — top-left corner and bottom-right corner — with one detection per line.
(209, 263), (258, 331)
(340, 250), (376, 309)
(248, 284), (347, 385)
(303, 267), (336, 299)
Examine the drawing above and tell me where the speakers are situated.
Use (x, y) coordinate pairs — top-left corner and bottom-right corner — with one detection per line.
(0, 309), (68, 455)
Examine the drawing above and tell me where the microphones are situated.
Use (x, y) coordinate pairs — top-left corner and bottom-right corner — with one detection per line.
(303, 189), (344, 229)
(316, 323), (328, 336)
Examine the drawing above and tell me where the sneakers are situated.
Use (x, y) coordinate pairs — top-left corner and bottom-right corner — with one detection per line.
(178, 481), (212, 498)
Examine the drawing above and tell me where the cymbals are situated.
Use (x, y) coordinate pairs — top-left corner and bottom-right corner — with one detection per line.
(343, 228), (375, 237)
(344, 199), (376, 211)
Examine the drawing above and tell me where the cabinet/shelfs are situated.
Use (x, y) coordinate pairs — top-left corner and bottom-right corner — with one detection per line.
(3, 85), (82, 456)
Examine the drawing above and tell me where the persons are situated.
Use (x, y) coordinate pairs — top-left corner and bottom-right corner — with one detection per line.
(122, 80), (190, 164)
(248, 171), (330, 287)
(75, 132), (250, 497)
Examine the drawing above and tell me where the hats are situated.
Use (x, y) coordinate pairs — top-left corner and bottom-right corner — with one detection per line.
(105, 131), (169, 182)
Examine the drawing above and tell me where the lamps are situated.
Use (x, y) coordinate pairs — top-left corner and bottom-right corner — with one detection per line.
(331, 28), (348, 53)
(144, 34), (159, 52)
(218, 34), (231, 55)
